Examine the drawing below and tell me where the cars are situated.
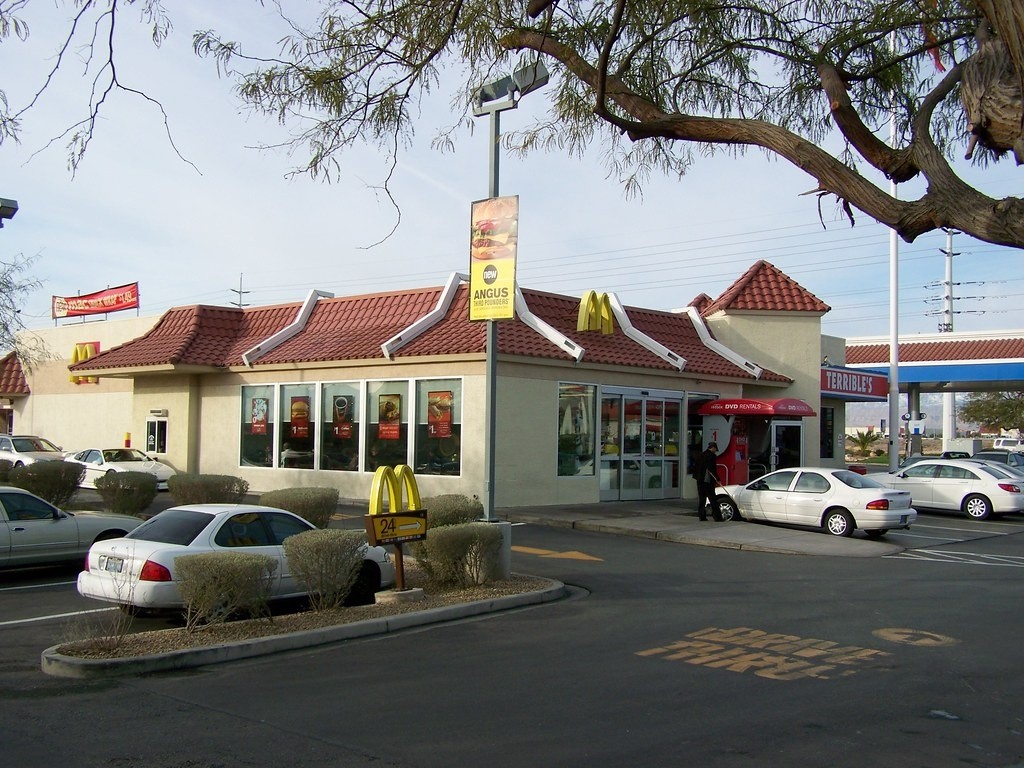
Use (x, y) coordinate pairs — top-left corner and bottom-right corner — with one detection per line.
(970, 447), (1024, 473)
(75, 503), (397, 611)
(706, 466), (918, 535)
(0, 434), (67, 467)
(862, 459), (1024, 519)
(576, 453), (663, 490)
(0, 486), (152, 568)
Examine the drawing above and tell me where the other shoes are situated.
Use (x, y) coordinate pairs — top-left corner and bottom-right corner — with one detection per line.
(699, 518), (709, 521)
(715, 519), (726, 522)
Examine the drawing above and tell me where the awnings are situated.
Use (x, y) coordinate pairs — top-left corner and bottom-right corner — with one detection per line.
(697, 398), (817, 417)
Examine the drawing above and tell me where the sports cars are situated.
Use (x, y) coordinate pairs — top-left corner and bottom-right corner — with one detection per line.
(62, 446), (178, 493)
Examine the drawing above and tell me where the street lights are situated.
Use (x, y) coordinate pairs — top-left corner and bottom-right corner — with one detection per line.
(469, 60), (549, 519)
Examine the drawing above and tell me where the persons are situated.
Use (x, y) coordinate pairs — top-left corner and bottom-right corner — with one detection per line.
(92, 452), (113, 465)
(692, 442), (726, 522)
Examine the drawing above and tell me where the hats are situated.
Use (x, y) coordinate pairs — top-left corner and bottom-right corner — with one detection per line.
(708, 442), (719, 451)
(283, 442), (290, 450)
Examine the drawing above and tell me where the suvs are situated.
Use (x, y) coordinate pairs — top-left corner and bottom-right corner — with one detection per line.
(993, 437), (1024, 452)
(894, 451), (970, 475)
